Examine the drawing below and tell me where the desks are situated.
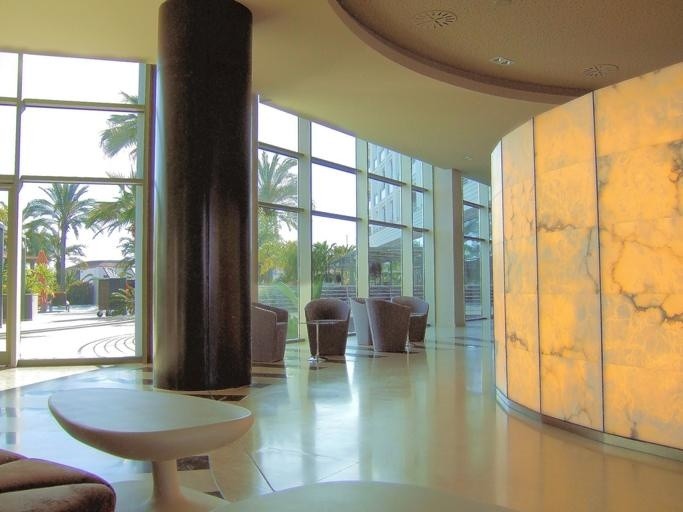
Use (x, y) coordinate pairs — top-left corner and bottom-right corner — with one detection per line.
(296, 319), (346, 370)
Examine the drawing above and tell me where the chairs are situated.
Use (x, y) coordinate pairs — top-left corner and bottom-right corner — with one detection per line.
(48, 389), (254, 512)
(304, 299), (350, 356)
(350, 297), (373, 346)
(0, 448), (116, 512)
(251, 302), (288, 363)
(392, 295), (429, 342)
(364, 298), (411, 353)
